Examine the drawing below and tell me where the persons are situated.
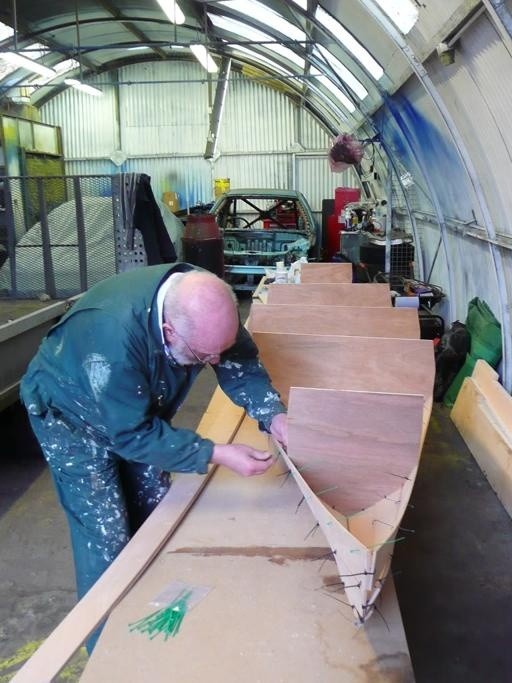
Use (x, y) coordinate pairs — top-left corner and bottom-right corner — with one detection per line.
(18, 260), (288, 658)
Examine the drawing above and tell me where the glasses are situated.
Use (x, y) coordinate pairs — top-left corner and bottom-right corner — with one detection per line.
(178, 333), (217, 366)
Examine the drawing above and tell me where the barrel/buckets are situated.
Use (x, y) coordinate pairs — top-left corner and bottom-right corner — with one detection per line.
(181, 216), (224, 281)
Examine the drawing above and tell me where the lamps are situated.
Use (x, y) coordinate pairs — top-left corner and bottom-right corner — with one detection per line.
(1, 0), (104, 103)
(155, 0), (221, 74)
